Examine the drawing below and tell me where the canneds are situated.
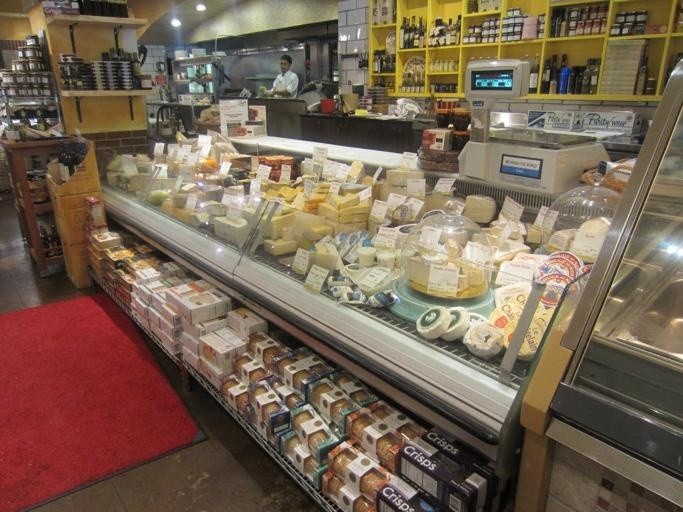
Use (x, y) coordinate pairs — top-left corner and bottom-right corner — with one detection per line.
(0, 36), (56, 100)
(568, 6), (609, 36)
(462, 7), (545, 43)
(610, 10), (647, 36)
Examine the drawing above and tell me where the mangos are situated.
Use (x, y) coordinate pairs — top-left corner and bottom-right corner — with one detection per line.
(147, 190), (169, 204)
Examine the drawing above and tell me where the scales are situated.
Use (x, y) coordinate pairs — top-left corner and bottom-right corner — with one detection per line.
(458, 57), (614, 195)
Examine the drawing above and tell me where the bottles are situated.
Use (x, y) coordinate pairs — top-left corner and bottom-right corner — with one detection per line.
(675, 3), (683, 33)
(0, 33), (52, 97)
(40, 224), (63, 259)
(19, 106), (46, 132)
(399, 4), (656, 96)
(371, 48), (386, 73)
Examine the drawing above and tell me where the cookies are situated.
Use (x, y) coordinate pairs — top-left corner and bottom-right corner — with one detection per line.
(224, 334), (419, 512)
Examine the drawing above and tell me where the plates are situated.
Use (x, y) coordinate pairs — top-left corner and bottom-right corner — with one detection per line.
(174, 48), (207, 59)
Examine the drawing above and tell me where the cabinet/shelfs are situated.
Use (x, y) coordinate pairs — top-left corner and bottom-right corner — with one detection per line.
(98, 141), (612, 466)
(367, 1), (683, 101)
(46, 13), (154, 99)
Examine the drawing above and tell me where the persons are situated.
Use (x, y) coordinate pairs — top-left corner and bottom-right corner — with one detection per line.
(259, 54), (299, 99)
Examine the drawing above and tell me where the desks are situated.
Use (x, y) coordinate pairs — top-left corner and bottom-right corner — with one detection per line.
(1, 135), (89, 279)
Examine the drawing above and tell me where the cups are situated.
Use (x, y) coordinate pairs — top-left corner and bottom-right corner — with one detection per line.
(356, 246), (377, 266)
(319, 97), (337, 114)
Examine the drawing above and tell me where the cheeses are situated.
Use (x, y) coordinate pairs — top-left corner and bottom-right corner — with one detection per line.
(160, 156), (495, 257)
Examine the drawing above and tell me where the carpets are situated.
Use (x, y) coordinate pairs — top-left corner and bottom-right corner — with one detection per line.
(1, 294), (209, 510)
